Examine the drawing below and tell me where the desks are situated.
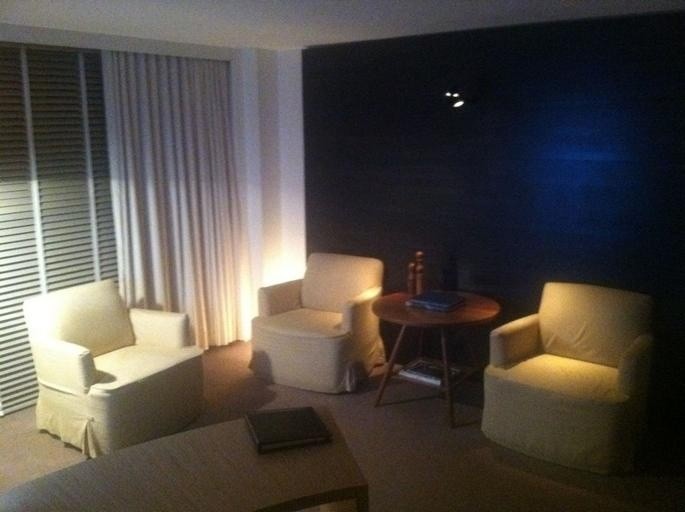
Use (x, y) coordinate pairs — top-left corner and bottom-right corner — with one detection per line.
(0, 406), (368, 512)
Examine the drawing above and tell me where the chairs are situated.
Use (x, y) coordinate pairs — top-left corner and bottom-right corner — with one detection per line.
(479, 282), (655, 478)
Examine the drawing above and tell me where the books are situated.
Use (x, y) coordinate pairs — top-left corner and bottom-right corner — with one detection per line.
(243, 406), (333, 456)
(409, 290), (464, 308)
(398, 361), (457, 386)
(405, 298), (466, 313)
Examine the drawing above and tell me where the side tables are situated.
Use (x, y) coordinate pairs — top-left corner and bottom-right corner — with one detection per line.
(371, 287), (509, 429)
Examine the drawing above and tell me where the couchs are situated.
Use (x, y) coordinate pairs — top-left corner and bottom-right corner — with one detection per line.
(23, 277), (206, 458)
(246, 251), (384, 395)
